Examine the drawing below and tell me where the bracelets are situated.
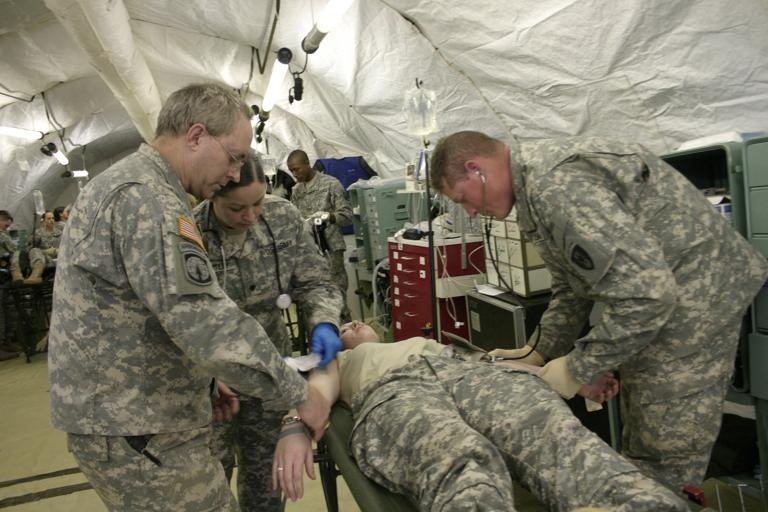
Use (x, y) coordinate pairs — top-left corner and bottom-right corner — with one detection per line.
(275, 427), (318, 441)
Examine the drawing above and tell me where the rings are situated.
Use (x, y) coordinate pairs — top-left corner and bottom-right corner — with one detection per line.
(276, 467), (284, 471)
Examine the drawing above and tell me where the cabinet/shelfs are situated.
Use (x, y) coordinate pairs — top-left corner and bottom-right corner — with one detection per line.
(654, 139), (767, 510)
(384, 232), (481, 345)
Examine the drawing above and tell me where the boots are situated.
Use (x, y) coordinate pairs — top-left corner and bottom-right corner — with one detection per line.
(10, 259), (45, 285)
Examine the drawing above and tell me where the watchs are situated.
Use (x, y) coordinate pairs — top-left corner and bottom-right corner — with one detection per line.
(278, 415), (317, 437)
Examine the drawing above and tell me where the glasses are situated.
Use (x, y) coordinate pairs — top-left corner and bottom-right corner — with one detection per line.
(205, 126), (246, 173)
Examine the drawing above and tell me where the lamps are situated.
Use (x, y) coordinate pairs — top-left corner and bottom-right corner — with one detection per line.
(243, 0), (355, 146)
(0, 92), (89, 178)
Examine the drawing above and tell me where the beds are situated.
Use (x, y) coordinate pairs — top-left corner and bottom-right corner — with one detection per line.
(315, 403), (712, 512)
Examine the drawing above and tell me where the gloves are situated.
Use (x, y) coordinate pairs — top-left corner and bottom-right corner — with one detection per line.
(307, 323), (344, 367)
(488, 343), (545, 368)
(536, 356), (583, 402)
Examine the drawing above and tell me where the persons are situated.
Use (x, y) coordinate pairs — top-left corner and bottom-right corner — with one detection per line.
(53, 205), (68, 233)
(269, 317), (695, 509)
(189, 146), (345, 512)
(31, 208), (63, 251)
(0, 209), (20, 364)
(44, 79), (331, 512)
(425, 130), (768, 498)
(7, 229), (52, 287)
(284, 149), (354, 327)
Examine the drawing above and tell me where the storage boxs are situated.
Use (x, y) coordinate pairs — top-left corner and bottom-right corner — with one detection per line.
(482, 208), (550, 295)
(463, 290), (609, 442)
